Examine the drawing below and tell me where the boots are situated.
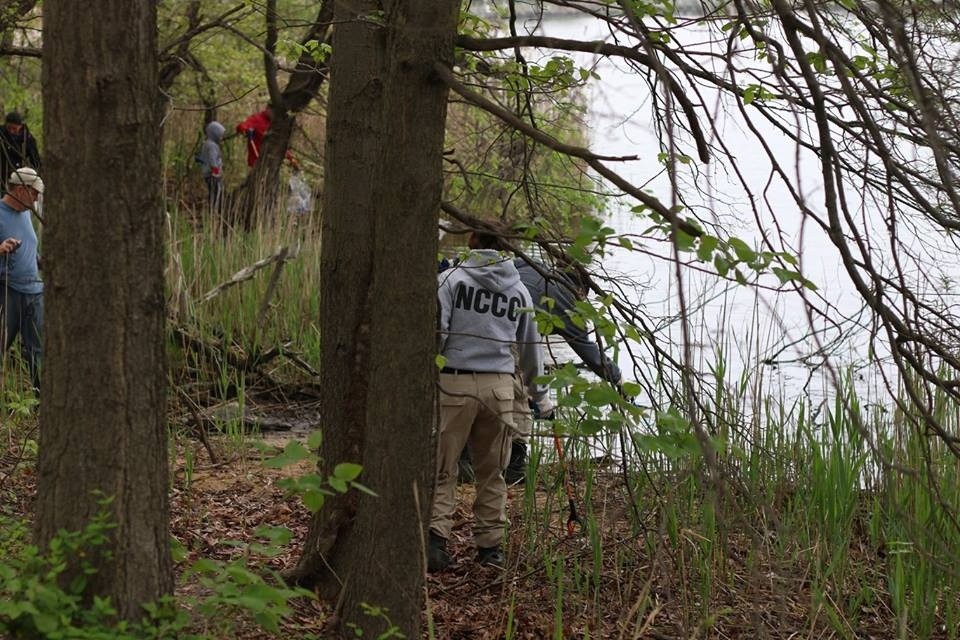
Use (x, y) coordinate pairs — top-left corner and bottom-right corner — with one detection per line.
(501, 440), (527, 485)
(456, 442), (475, 485)
(426, 529), (453, 574)
(472, 547), (506, 571)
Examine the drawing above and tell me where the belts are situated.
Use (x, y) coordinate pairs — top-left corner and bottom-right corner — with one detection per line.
(441, 366), (490, 375)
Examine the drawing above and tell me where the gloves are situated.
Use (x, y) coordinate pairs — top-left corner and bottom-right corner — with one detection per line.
(245, 128), (254, 138)
(213, 167), (222, 180)
(617, 375), (636, 400)
(535, 395), (554, 418)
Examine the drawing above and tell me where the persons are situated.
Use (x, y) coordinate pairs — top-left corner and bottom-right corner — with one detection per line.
(427, 223), (621, 572)
(236, 100), (295, 208)
(199, 122), (225, 215)
(0, 114), (45, 415)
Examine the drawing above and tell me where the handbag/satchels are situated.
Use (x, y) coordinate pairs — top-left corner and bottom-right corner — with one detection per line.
(288, 171), (309, 213)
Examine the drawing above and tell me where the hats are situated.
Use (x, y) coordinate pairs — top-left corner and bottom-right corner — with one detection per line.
(10, 168), (44, 192)
(468, 220), (511, 251)
(6, 112), (22, 125)
